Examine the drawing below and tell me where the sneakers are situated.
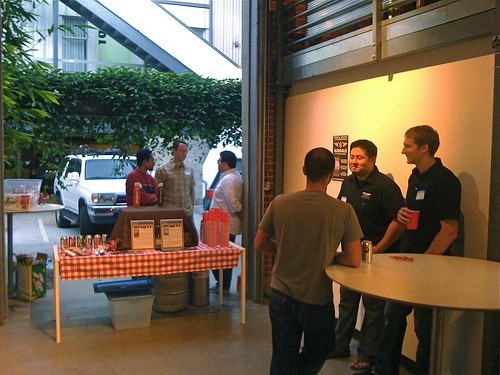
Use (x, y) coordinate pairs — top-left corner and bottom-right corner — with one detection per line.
(208, 284), (228, 294)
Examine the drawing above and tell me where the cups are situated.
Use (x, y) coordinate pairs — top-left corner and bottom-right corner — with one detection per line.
(406, 210), (420, 230)
(208, 189), (214, 199)
(200, 206), (231, 248)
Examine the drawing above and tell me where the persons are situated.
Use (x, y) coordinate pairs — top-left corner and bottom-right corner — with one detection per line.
(210, 151), (242, 243)
(253, 147), (364, 375)
(126, 140), (196, 224)
(324, 140), (407, 369)
(353, 125), (461, 375)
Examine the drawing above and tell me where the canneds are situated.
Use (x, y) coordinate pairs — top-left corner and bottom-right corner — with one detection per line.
(361, 240), (373, 263)
(69, 236), (76, 247)
(85, 235), (92, 248)
(61, 236), (68, 249)
(77, 235), (84, 247)
(102, 234), (108, 246)
(94, 235), (101, 248)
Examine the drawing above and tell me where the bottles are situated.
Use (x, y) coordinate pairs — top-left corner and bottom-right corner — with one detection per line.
(133, 182), (141, 208)
(157, 182), (164, 207)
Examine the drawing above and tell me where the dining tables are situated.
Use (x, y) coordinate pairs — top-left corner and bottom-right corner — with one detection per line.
(53, 240), (246, 344)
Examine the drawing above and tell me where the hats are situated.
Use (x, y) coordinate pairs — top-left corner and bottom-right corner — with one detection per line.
(136, 149), (152, 157)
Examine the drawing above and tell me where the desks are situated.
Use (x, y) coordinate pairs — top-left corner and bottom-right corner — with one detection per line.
(326, 253), (500, 374)
(108, 204), (199, 249)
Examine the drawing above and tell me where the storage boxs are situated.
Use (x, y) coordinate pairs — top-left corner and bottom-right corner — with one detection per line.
(15, 252), (48, 302)
(149, 271), (192, 312)
(3, 178), (43, 212)
(104, 291), (155, 331)
(191, 269), (210, 307)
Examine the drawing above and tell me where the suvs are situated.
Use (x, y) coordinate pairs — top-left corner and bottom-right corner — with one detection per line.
(53, 149), (151, 240)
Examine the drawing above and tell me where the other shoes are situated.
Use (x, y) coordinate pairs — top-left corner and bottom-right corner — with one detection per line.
(350, 358), (372, 371)
